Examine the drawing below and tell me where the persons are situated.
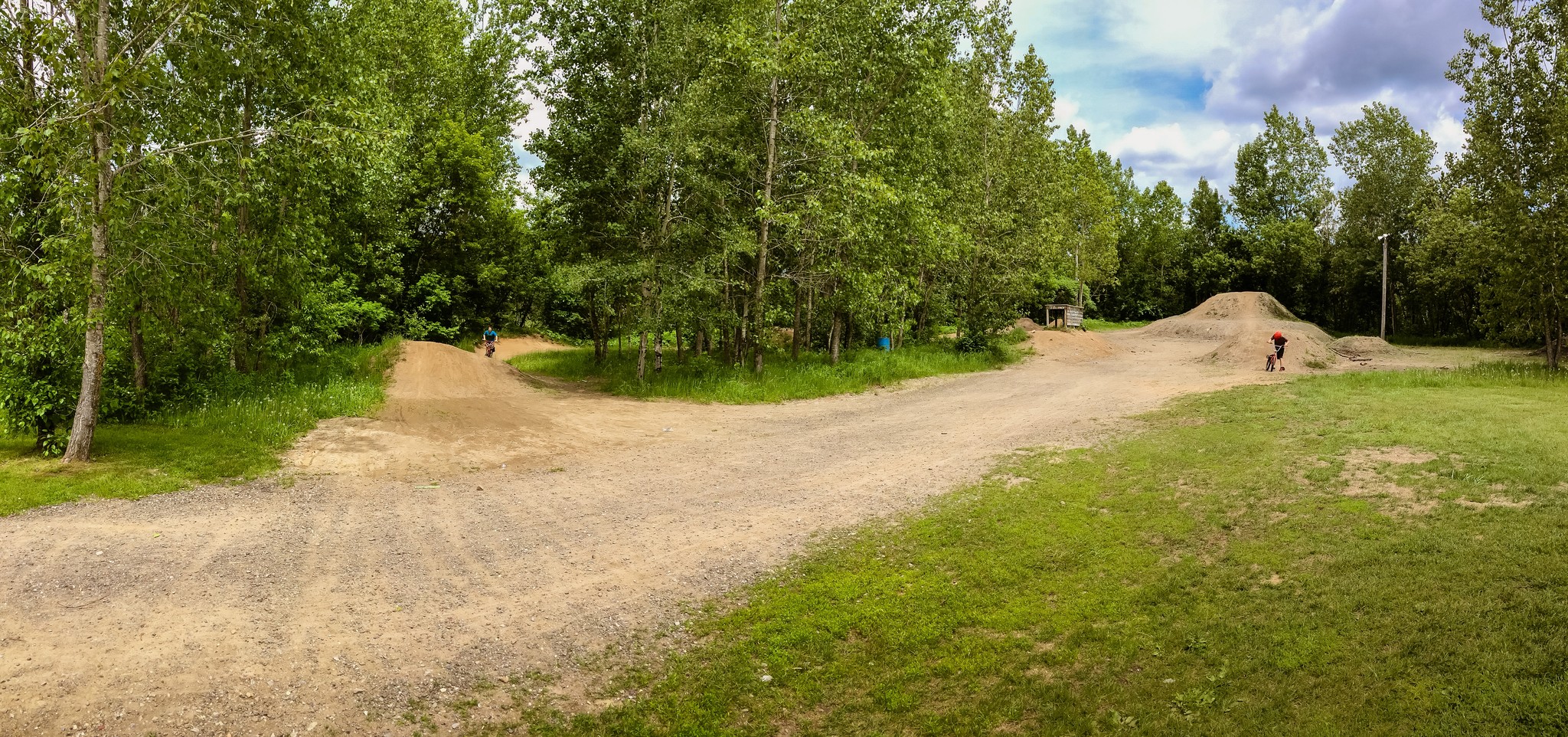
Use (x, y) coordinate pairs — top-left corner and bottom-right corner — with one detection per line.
(1267, 331), (1290, 371)
(483, 326), (498, 356)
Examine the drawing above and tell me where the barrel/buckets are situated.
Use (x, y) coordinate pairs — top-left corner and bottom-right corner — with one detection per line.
(877, 338), (890, 350)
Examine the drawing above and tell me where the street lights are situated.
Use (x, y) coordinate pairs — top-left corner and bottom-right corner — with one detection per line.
(1378, 233), (1390, 340)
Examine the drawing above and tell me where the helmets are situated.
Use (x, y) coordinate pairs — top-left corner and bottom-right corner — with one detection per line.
(1274, 331), (1281, 339)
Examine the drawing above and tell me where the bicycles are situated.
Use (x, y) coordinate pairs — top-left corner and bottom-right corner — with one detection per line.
(1266, 341), (1288, 372)
(482, 339), (499, 358)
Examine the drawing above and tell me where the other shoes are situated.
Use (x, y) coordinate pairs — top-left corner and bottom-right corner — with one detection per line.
(1279, 368), (1283, 372)
(493, 347), (495, 352)
